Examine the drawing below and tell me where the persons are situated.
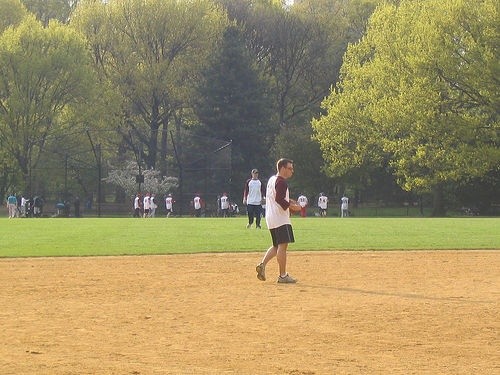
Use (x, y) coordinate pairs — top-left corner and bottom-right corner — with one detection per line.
(243, 168), (266, 229)
(256, 158), (302, 283)
(6, 188), (352, 220)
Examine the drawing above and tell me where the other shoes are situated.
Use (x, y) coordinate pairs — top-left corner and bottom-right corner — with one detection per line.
(246, 223), (251, 229)
(256, 226), (261, 230)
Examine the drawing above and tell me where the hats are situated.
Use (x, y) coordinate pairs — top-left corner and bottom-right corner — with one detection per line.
(252, 169), (258, 174)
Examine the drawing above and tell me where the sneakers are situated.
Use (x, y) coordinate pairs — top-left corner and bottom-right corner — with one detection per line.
(277, 273), (297, 283)
(256, 263), (266, 280)
